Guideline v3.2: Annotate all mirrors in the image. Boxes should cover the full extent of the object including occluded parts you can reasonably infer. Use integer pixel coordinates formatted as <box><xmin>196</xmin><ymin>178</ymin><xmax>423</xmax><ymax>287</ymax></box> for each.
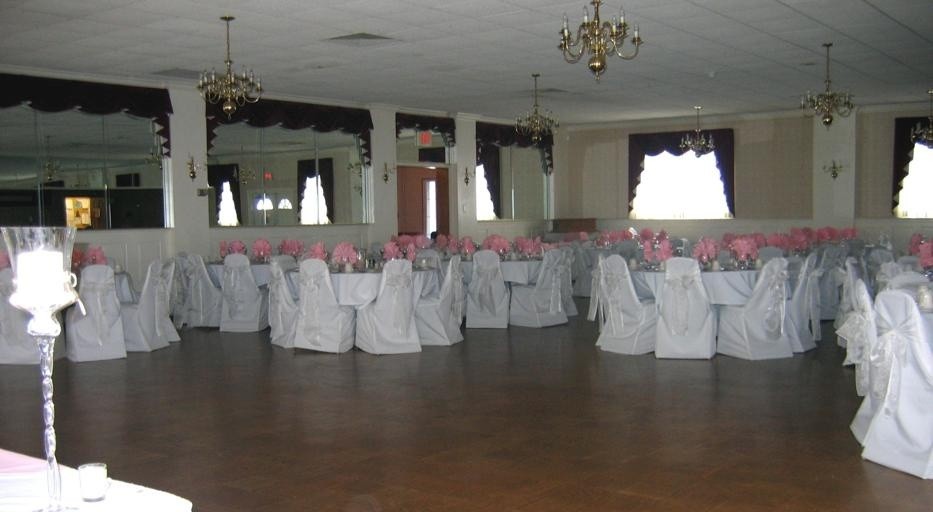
<box><xmin>473</xmin><ymin>118</ymin><xmax>551</xmax><ymax>220</ymax></box>
<box><xmin>203</xmin><ymin>90</ymin><xmax>377</xmax><ymax>229</ymax></box>
<box><xmin>0</xmin><ymin>71</ymin><xmax>174</xmax><ymax>233</ymax></box>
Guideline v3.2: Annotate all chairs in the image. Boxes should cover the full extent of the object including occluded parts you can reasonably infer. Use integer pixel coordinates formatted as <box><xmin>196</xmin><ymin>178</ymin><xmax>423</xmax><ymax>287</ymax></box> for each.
<box><xmin>175</xmin><ymin>234</ymin><xmax>589</xmax><ymax>353</ymax></box>
<box><xmin>585</xmin><ymin>224</ymin><xmax>855</xmax><ymax>361</ymax></box>
<box><xmin>833</xmin><ymin>232</ymin><xmax>933</xmax><ymax>479</ymax></box>
<box><xmin>1</xmin><ymin>246</ymin><xmax>177</xmax><ymax>365</ymax></box>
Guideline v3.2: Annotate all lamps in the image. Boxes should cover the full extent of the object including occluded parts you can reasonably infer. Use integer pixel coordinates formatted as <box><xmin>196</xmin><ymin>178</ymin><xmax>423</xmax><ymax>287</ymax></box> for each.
<box><xmin>796</xmin><ymin>36</ymin><xmax>863</xmax><ymax>136</ymax></box>
<box><xmin>672</xmin><ymin>103</ymin><xmax>722</xmax><ymax>161</ymax></box>
<box><xmin>188</xmin><ymin>12</ymin><xmax>268</xmax><ymax>125</ymax></box>
<box><xmin>40</xmin><ymin>134</ymin><xmax>60</xmax><ymax>183</ymax></box>
<box><xmin>255</xmin><ymin>189</ymin><xmax>275</xmax><ymax>226</ymax></box>
<box><xmin>546</xmin><ymin>2</ymin><xmax>648</xmax><ymax>93</ymax></box>
<box><xmin>520</xmin><ymin>72</ymin><xmax>564</xmax><ymax>146</ymax></box>
